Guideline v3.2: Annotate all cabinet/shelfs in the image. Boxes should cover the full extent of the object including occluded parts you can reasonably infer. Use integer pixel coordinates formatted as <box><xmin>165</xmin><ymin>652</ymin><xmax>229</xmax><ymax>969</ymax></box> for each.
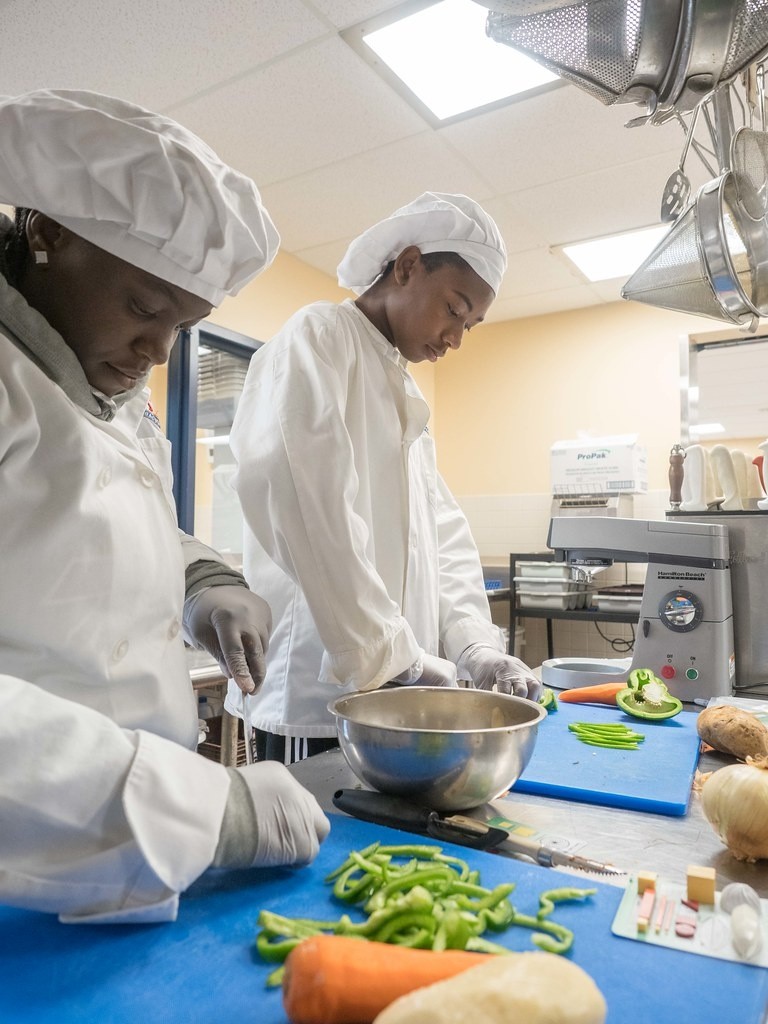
<box><xmin>509</xmin><ymin>552</ymin><xmax>640</xmax><ymax>658</ymax></box>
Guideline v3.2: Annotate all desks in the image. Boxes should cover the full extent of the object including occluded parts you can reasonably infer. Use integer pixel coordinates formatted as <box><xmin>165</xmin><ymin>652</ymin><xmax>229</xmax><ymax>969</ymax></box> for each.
<box><xmin>0</xmin><ymin>646</ymin><xmax>768</xmax><ymax>1024</ymax></box>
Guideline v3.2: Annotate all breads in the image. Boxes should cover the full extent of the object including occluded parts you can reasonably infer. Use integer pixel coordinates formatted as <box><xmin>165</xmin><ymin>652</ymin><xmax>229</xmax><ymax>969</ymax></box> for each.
<box><xmin>696</xmin><ymin>704</ymin><xmax>768</xmax><ymax>760</ymax></box>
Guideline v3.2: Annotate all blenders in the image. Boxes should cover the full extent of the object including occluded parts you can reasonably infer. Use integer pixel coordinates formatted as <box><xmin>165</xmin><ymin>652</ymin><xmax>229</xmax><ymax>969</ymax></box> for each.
<box><xmin>541</xmin><ymin>516</ymin><xmax>736</xmax><ymax>703</ymax></box>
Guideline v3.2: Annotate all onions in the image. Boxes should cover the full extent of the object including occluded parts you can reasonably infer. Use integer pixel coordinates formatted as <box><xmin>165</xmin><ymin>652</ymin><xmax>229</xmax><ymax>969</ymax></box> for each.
<box><xmin>700</xmin><ymin>754</ymin><xmax>768</xmax><ymax>862</ymax></box>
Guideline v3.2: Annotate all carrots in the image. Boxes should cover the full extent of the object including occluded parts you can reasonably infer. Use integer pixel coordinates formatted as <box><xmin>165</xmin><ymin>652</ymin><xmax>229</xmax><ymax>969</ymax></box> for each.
<box><xmin>282</xmin><ymin>935</ymin><xmax>516</xmax><ymax>1024</ymax></box>
<box><xmin>558</xmin><ymin>681</ymin><xmax>629</xmax><ymax>706</ymax></box>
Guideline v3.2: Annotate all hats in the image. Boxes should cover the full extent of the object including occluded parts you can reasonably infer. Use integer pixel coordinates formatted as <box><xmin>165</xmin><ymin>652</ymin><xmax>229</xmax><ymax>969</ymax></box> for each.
<box><xmin>336</xmin><ymin>191</ymin><xmax>509</xmax><ymax>299</ymax></box>
<box><xmin>0</xmin><ymin>88</ymin><xmax>280</xmax><ymax>308</ymax></box>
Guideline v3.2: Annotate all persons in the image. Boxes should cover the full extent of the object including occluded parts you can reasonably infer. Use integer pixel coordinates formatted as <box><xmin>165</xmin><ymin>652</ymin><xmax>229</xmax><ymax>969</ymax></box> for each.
<box><xmin>0</xmin><ymin>89</ymin><xmax>331</xmax><ymax>925</ymax></box>
<box><xmin>224</xmin><ymin>188</ymin><xmax>542</xmax><ymax>764</ymax></box>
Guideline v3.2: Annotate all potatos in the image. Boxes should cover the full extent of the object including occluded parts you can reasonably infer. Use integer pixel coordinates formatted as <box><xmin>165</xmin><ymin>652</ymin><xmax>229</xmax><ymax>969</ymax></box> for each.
<box><xmin>373</xmin><ymin>949</ymin><xmax>607</xmax><ymax>1024</ymax></box>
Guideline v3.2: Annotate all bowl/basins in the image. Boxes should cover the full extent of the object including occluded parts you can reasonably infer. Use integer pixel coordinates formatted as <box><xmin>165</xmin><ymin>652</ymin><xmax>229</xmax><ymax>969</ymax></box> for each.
<box><xmin>326</xmin><ymin>685</ymin><xmax>547</xmax><ymax>813</ymax></box>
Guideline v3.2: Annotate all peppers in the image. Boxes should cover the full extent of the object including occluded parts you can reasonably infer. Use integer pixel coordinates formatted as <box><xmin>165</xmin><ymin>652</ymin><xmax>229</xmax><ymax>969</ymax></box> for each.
<box><xmin>255</xmin><ymin>840</ymin><xmax>598</xmax><ymax>987</ymax></box>
<box><xmin>536</xmin><ymin>687</ymin><xmax>558</xmax><ymax>711</ymax></box>
<box><xmin>616</xmin><ymin>669</ymin><xmax>683</xmax><ymax>720</ymax></box>
<box><xmin>567</xmin><ymin>722</ymin><xmax>646</xmax><ymax>750</ymax></box>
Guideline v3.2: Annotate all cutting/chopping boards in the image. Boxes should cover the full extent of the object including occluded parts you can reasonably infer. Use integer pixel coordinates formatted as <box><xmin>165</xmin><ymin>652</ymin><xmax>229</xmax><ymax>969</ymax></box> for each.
<box><xmin>0</xmin><ymin>810</ymin><xmax>768</xmax><ymax>1024</ymax></box>
<box><xmin>508</xmin><ymin>693</ymin><xmax>701</xmax><ymax>818</ymax></box>
